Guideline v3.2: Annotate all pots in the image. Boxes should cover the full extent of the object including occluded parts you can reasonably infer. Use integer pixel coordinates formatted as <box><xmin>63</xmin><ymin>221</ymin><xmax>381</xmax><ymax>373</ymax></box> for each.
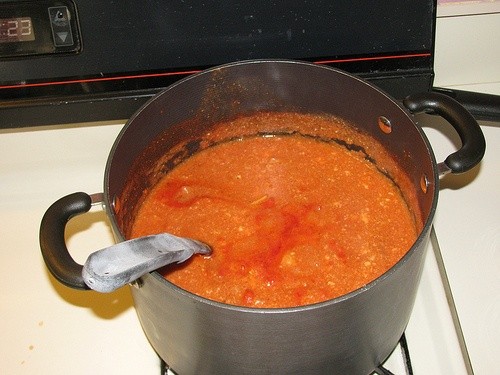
<box><xmin>38</xmin><ymin>55</ymin><xmax>487</xmax><ymax>374</ymax></box>
